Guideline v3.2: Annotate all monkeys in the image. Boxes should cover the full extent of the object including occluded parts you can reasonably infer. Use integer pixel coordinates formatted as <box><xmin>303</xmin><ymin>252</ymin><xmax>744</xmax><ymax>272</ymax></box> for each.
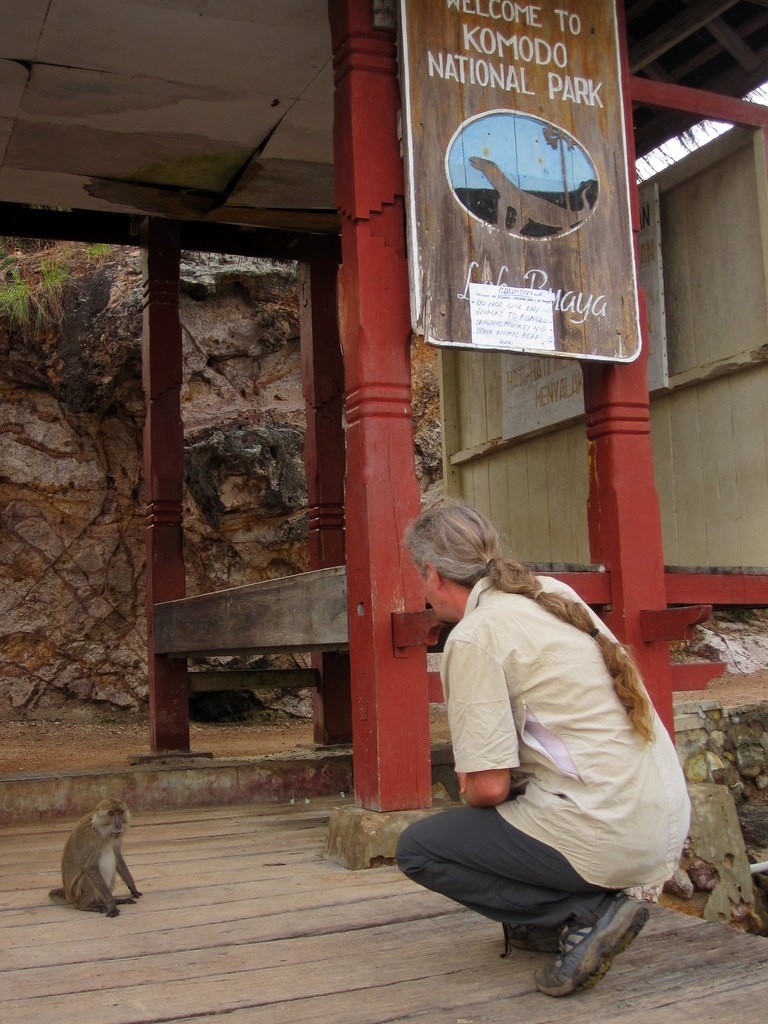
<box><xmin>49</xmin><ymin>798</ymin><xmax>143</xmax><ymax>918</ymax></box>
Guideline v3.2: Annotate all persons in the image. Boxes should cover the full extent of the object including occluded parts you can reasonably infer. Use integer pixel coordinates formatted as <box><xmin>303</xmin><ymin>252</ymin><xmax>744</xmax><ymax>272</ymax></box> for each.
<box><xmin>395</xmin><ymin>502</ymin><xmax>691</xmax><ymax>997</ymax></box>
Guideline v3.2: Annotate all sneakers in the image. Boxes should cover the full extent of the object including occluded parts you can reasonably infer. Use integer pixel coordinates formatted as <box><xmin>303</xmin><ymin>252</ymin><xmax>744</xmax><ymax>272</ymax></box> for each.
<box><xmin>508</xmin><ymin>920</ymin><xmax>563</xmax><ymax>952</ymax></box>
<box><xmin>533</xmin><ymin>891</ymin><xmax>650</xmax><ymax>997</ymax></box>
